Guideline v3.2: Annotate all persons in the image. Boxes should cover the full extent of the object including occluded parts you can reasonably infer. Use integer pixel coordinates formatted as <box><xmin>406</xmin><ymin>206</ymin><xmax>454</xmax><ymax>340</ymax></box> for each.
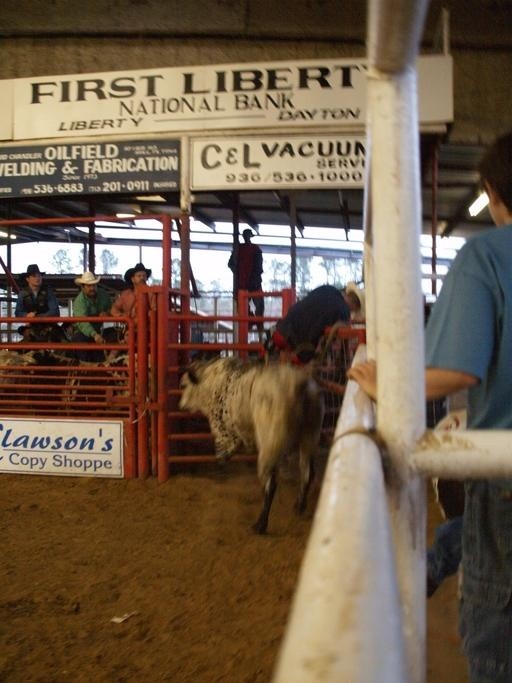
<box><xmin>345</xmin><ymin>130</ymin><xmax>512</xmax><ymax>683</ymax></box>
<box><xmin>272</xmin><ymin>281</ymin><xmax>364</xmax><ymax>367</ymax></box>
<box><xmin>110</xmin><ymin>263</ymin><xmax>156</xmax><ymax>315</ymax></box>
<box><xmin>228</xmin><ymin>229</ymin><xmax>264</xmax><ymax>329</ymax></box>
<box><xmin>14</xmin><ymin>265</ymin><xmax>60</xmax><ymax>317</ymax></box>
<box><xmin>72</xmin><ymin>272</ymin><xmax>112</xmax><ymax>344</ymax></box>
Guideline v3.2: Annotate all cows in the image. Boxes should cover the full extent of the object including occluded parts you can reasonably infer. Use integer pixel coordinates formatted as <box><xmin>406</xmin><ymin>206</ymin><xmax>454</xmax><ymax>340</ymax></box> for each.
<box><xmin>23</xmin><ymin>350</ymin><xmax>149</xmax><ymax>416</ymax></box>
<box><xmin>178</xmin><ymin>322</ymin><xmax>345</xmax><ymax>538</ymax></box>
<box><xmin>0</xmin><ymin>350</ymin><xmax>23</xmax><ymax>385</ymax></box>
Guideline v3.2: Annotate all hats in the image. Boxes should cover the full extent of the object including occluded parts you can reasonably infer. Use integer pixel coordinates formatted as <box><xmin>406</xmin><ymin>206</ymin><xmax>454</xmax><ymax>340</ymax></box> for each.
<box><xmin>346</xmin><ymin>282</ymin><xmax>366</xmax><ymax>322</ymax></box>
<box><xmin>124</xmin><ymin>262</ymin><xmax>152</xmax><ymax>283</ymax></box>
<box><xmin>22</xmin><ymin>263</ymin><xmax>45</xmax><ymax>278</ymax></box>
<box><xmin>75</xmin><ymin>271</ymin><xmax>101</xmax><ymax>287</ymax></box>
